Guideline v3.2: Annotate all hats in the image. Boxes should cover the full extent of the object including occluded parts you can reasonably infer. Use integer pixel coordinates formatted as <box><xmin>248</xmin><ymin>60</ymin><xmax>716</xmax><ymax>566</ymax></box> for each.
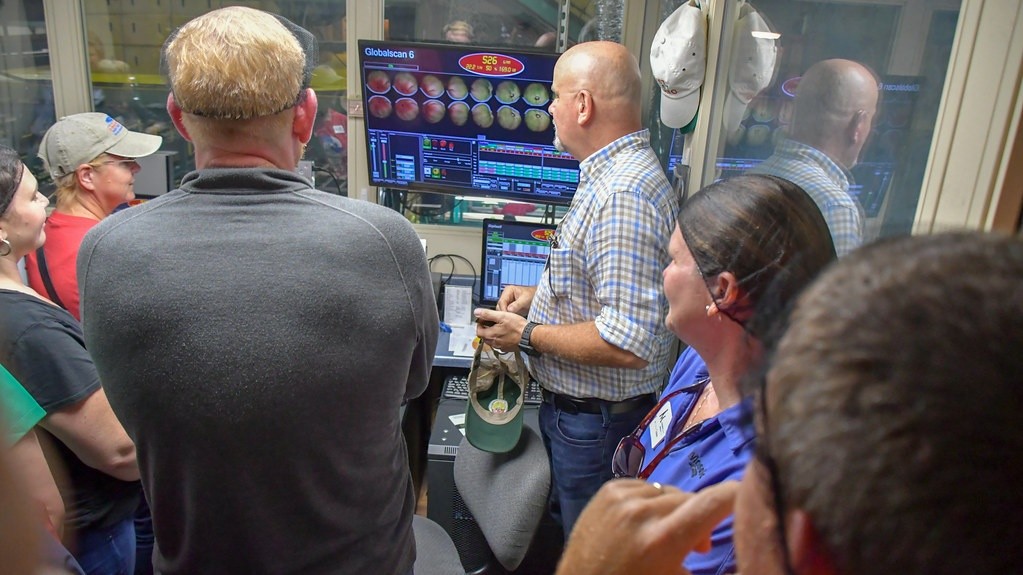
<box><xmin>465</xmin><ymin>337</ymin><xmax>530</xmax><ymax>454</ymax></box>
<box><xmin>649</xmin><ymin>0</ymin><xmax>708</xmax><ymax>135</ymax></box>
<box><xmin>37</xmin><ymin>113</ymin><xmax>163</xmax><ymax>179</ymax></box>
<box><xmin>723</xmin><ymin>2</ymin><xmax>777</xmax><ymax>131</ymax></box>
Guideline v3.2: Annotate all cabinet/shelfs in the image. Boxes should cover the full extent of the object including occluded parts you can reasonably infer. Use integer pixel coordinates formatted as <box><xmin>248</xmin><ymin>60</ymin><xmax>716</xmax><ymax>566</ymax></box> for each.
<box><xmin>82</xmin><ymin>0</ymin><xmax>222</xmax><ymax>73</ymax></box>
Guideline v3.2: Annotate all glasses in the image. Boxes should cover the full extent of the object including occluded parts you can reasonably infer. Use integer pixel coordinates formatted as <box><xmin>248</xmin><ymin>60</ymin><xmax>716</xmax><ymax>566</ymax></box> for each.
<box><xmin>57</xmin><ymin>157</ymin><xmax>136</xmax><ymax>179</ymax></box>
<box><xmin>612</xmin><ymin>378</ymin><xmax>707</xmax><ymax>480</ymax></box>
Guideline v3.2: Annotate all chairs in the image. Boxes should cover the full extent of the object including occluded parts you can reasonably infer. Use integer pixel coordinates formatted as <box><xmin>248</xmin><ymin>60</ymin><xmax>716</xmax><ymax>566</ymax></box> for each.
<box><xmin>411</xmin><ymin>424</ymin><xmax>551</xmax><ymax>575</ymax></box>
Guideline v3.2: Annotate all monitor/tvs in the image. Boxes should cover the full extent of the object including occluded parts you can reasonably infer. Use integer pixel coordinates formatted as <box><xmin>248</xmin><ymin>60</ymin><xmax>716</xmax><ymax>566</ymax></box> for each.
<box><xmin>666</xmin><ymin>71</ymin><xmax>927</xmax><ymax>221</ymax></box>
<box><xmin>358</xmin><ymin>39</ymin><xmax>580</xmax><ymax>205</ymax></box>
<box><xmin>480</xmin><ymin>218</ymin><xmax>558</xmax><ymax>308</ymax></box>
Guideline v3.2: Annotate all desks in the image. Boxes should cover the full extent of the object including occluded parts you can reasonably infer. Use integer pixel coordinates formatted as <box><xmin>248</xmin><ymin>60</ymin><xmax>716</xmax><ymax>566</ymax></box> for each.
<box><xmin>0</xmin><ymin>64</ymin><xmax>170</xmax><ymax>89</ymax></box>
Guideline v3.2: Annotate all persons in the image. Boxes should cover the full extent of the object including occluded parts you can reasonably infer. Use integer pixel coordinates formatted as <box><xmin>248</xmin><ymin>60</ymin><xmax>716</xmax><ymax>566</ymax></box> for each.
<box><xmin>78</xmin><ymin>5</ymin><xmax>440</xmax><ymax>575</ymax></box>
<box><xmin>557</xmin><ymin>229</ymin><xmax>1023</xmax><ymax>575</ymax></box>
<box><xmin>474</xmin><ymin>41</ymin><xmax>680</xmax><ymax>575</ymax></box>
<box><xmin>24</xmin><ymin>112</ymin><xmax>163</xmax><ymax>320</ymax></box>
<box><xmin>629</xmin><ymin>173</ymin><xmax>839</xmax><ymax>575</ymax></box>
<box><xmin>88</xmin><ymin>31</ymin><xmax>108</xmax><ymax>109</ymax></box>
<box><xmin>752</xmin><ymin>58</ymin><xmax>879</xmax><ymax>256</ymax></box>
<box><xmin>443</xmin><ymin>21</ymin><xmax>474</xmax><ymax>42</ymax></box>
<box><xmin>0</xmin><ymin>145</ymin><xmax>142</xmax><ymax>575</ymax></box>
<box><xmin>0</xmin><ymin>364</ymin><xmax>66</xmax><ymax>540</ymax></box>
<box><xmin>314</xmin><ymin>95</ymin><xmax>347</xmax><ymax>176</ymax></box>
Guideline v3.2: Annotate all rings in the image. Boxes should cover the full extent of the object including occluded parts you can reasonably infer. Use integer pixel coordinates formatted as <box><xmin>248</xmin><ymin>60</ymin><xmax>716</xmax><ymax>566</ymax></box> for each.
<box><xmin>653</xmin><ymin>482</ymin><xmax>665</xmax><ymax>495</ymax></box>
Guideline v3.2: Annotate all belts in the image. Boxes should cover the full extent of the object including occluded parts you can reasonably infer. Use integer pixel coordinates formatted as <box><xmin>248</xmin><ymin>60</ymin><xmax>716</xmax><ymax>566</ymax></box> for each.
<box><xmin>539</xmin><ymin>384</ymin><xmax>656</xmax><ymax>416</ymax></box>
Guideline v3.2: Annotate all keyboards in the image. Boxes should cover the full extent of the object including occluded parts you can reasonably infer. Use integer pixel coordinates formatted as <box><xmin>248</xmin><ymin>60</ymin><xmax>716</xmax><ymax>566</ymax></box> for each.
<box><xmin>443</xmin><ymin>377</ymin><xmax>543</xmax><ymax>404</ymax></box>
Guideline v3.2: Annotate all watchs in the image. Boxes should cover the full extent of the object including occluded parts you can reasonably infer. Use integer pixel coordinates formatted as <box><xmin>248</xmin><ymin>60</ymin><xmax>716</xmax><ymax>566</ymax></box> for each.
<box><xmin>518</xmin><ymin>321</ymin><xmax>543</xmax><ymax>357</ymax></box>
